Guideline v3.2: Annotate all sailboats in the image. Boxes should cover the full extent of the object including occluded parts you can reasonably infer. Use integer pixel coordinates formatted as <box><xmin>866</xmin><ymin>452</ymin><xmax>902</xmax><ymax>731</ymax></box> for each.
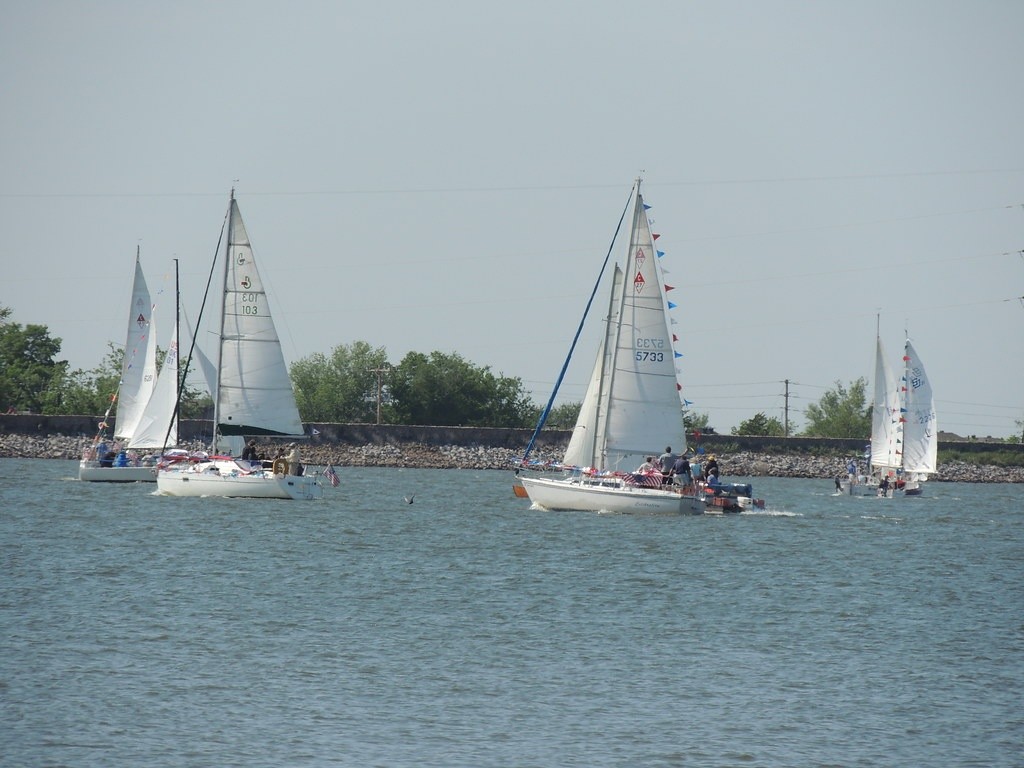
<box><xmin>515</xmin><ymin>173</ymin><xmax>765</xmax><ymax>518</ymax></box>
<box><xmin>835</xmin><ymin>314</ymin><xmax>939</xmax><ymax>497</ymax></box>
<box><xmin>77</xmin><ymin>243</ymin><xmax>217</xmax><ymax>480</ymax></box>
<box><xmin>155</xmin><ymin>188</ymin><xmax>319</xmax><ymax>499</ymax></box>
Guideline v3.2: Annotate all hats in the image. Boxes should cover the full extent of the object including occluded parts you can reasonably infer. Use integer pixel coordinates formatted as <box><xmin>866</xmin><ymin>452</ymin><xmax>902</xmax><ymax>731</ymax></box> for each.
<box><xmin>708</xmin><ymin>456</ymin><xmax>715</xmax><ymax>460</ymax></box>
<box><xmin>100</xmin><ymin>437</ymin><xmax>108</xmax><ymax>443</ymax></box>
<box><xmin>693</xmin><ymin>458</ymin><xmax>700</xmax><ymax>463</ymax></box>
<box><xmin>289</xmin><ymin>442</ymin><xmax>297</xmax><ymax>447</ymax></box>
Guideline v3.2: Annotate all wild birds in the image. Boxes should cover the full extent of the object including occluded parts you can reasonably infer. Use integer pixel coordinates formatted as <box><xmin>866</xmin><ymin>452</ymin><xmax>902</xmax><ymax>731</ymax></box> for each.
<box><xmin>404</xmin><ymin>495</ymin><xmax>414</xmax><ymax>504</ymax></box>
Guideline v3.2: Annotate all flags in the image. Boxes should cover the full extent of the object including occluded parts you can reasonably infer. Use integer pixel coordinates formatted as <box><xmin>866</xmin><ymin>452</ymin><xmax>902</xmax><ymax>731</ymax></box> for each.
<box><xmin>323</xmin><ymin>465</ymin><xmax>340</xmax><ymax>487</ymax></box>
<box><xmin>620</xmin><ymin>473</ymin><xmax>662</xmax><ymax>488</ymax></box>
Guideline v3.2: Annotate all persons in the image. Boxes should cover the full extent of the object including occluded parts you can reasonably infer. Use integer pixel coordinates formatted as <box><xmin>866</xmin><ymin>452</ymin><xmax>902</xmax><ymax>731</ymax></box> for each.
<box><xmin>637</xmin><ymin>447</ymin><xmax>719</xmax><ymax>495</ymax></box>
<box><xmin>0</xmin><ymin>432</ymin><xmax>1024</xmax><ymax>482</ymax></box>
<box><xmin>833</xmin><ymin>460</ymin><xmax>903</xmax><ymax>496</ymax></box>
